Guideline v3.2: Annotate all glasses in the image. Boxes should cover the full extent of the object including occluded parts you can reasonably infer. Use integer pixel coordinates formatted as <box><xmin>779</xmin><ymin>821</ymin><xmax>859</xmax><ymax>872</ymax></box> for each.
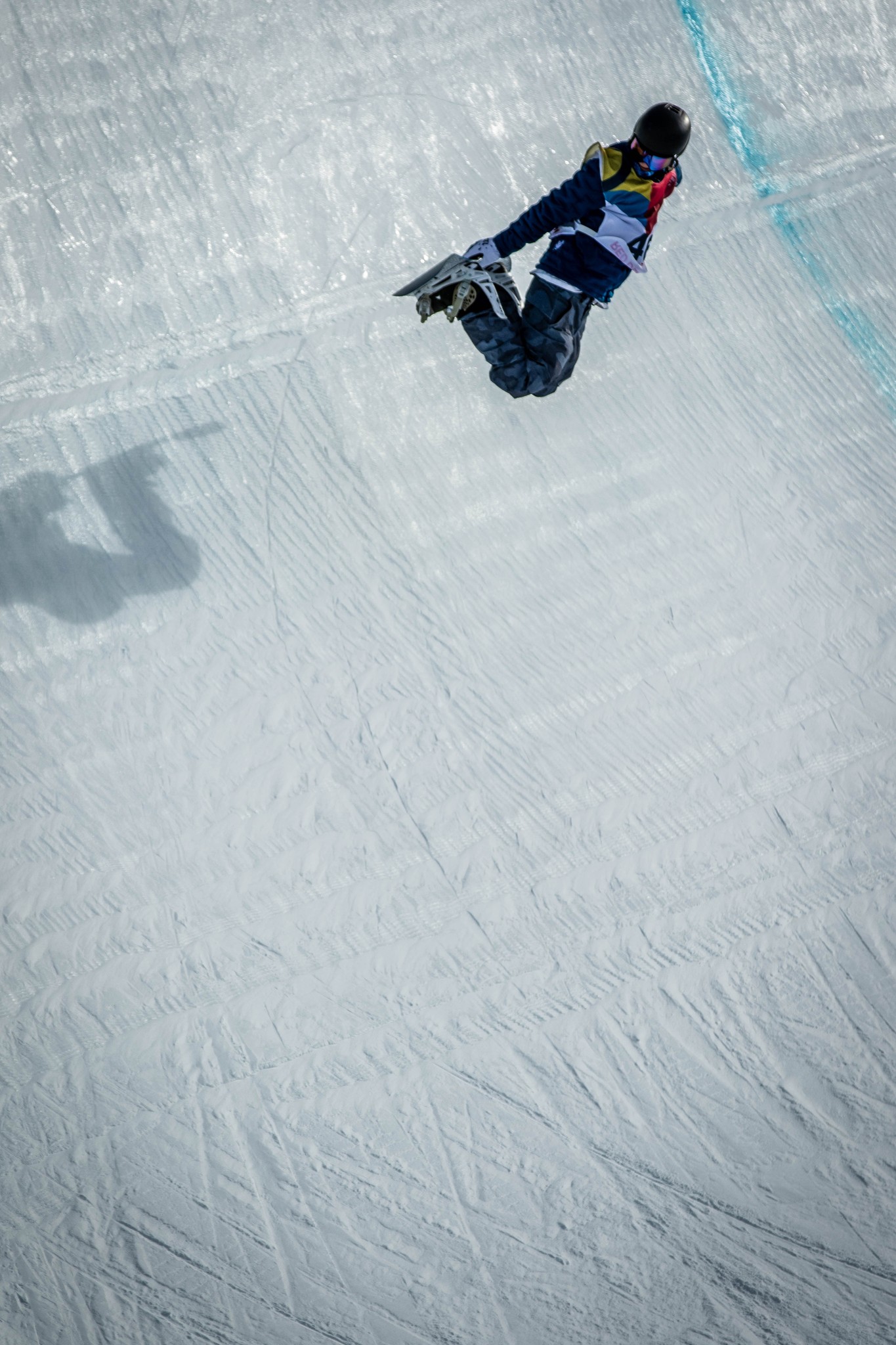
<box><xmin>629</xmin><ymin>133</ymin><xmax>674</xmax><ymax>171</ymax></box>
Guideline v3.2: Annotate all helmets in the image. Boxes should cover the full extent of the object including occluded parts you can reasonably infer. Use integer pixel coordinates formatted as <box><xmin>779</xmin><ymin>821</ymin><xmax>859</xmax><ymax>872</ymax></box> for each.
<box><xmin>627</xmin><ymin>102</ymin><xmax>691</xmax><ymax>180</ymax></box>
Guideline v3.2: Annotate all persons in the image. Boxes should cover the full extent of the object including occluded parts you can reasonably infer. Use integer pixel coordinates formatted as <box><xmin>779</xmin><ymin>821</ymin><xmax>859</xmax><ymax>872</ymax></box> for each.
<box><xmin>416</xmin><ymin>102</ymin><xmax>691</xmax><ymax>399</ymax></box>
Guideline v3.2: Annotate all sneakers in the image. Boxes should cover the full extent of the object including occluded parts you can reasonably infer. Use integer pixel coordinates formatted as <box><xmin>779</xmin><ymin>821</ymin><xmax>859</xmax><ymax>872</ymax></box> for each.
<box><xmin>416</xmin><ymin>256</ymin><xmax>508</xmax><ymax>319</ymax></box>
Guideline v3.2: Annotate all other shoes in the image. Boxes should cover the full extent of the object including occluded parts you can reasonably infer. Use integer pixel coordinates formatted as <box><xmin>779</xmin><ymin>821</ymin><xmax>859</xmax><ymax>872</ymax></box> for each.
<box><xmin>482</xmin><ymin>261</ymin><xmax>522</xmax><ymax>320</ymax></box>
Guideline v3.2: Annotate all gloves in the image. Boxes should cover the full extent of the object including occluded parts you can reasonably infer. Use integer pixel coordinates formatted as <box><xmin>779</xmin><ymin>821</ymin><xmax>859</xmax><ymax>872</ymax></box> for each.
<box><xmin>464</xmin><ymin>237</ymin><xmax>500</xmax><ymax>268</ymax></box>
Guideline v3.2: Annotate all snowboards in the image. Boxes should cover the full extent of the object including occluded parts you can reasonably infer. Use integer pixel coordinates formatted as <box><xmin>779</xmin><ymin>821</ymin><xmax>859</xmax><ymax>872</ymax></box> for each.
<box><xmin>391</xmin><ymin>252</ymin><xmax>512</xmax><ymax>297</ymax></box>
